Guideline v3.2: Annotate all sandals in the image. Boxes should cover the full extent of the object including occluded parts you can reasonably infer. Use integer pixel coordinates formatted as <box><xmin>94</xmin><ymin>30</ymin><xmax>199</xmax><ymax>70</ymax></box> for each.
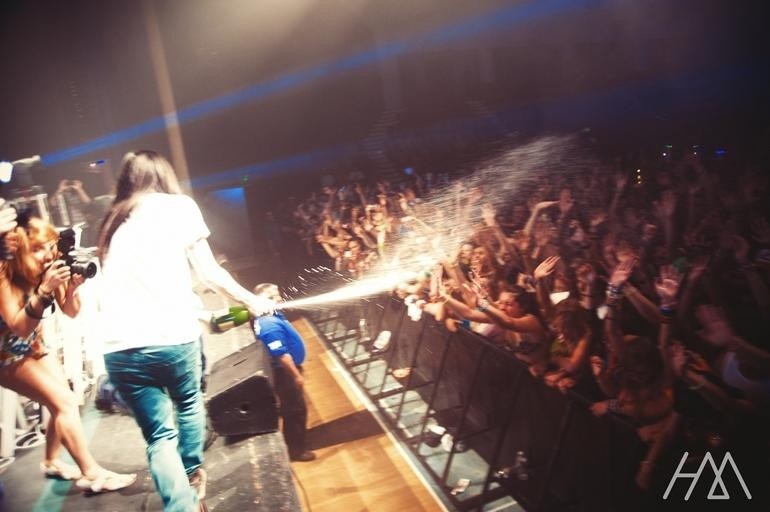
<box><xmin>39</xmin><ymin>459</ymin><xmax>78</xmax><ymax>481</ymax></box>
<box><xmin>77</xmin><ymin>468</ymin><xmax>139</xmax><ymax>492</ymax></box>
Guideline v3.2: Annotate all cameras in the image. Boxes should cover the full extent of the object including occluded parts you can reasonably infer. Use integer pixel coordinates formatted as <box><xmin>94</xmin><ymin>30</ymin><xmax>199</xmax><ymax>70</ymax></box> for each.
<box><xmin>56</xmin><ymin>229</ymin><xmax>97</xmax><ymax>278</ymax></box>
<box><xmin>10</xmin><ymin>201</ymin><xmax>32</xmax><ymax>226</ymax></box>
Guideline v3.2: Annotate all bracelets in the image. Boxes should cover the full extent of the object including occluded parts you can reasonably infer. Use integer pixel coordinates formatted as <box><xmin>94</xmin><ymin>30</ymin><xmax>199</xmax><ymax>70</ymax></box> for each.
<box><xmin>38</xmin><ymin>284</ymin><xmax>52</xmax><ymax>296</ymax></box>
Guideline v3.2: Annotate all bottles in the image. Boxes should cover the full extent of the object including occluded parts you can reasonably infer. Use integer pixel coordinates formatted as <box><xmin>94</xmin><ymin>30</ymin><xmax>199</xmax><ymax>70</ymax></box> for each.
<box><xmin>514</xmin><ymin>450</ymin><xmax>530</xmax><ymax>483</ymax></box>
<box><xmin>210</xmin><ymin>296</ymin><xmax>277</xmax><ymax>332</ymax></box>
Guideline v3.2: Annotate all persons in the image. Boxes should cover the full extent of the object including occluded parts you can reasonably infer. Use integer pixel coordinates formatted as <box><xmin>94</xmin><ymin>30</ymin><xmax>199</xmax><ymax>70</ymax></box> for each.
<box><xmin>0</xmin><ymin>197</ymin><xmax>22</xmax><ymax>262</ymax></box>
<box><xmin>46</xmin><ymin>177</ymin><xmax>93</xmax><ymax>229</ymax></box>
<box><xmin>1</xmin><ymin>215</ymin><xmax>140</xmax><ymax>497</ymax></box>
<box><xmin>248</xmin><ymin>100</ymin><xmax>770</xmax><ymax>490</ymax></box>
<box><xmin>85</xmin><ymin>147</ymin><xmax>278</xmax><ymax>512</ymax></box>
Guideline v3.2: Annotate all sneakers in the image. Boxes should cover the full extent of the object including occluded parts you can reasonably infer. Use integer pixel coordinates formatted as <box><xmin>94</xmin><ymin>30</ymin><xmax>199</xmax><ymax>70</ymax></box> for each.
<box><xmin>290</xmin><ymin>449</ymin><xmax>315</xmax><ymax>462</ymax></box>
<box><xmin>189</xmin><ymin>468</ymin><xmax>206</xmax><ymax>501</ymax></box>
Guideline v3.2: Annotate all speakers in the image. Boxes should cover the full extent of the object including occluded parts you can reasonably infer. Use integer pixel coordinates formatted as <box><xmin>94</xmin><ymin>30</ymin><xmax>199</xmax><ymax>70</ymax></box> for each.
<box><xmin>203</xmin><ymin>341</ymin><xmax>278</xmax><ymax>434</ymax></box>
<box><xmin>203</xmin><ymin>444</ymin><xmax>301</xmax><ymax>512</ymax></box>
